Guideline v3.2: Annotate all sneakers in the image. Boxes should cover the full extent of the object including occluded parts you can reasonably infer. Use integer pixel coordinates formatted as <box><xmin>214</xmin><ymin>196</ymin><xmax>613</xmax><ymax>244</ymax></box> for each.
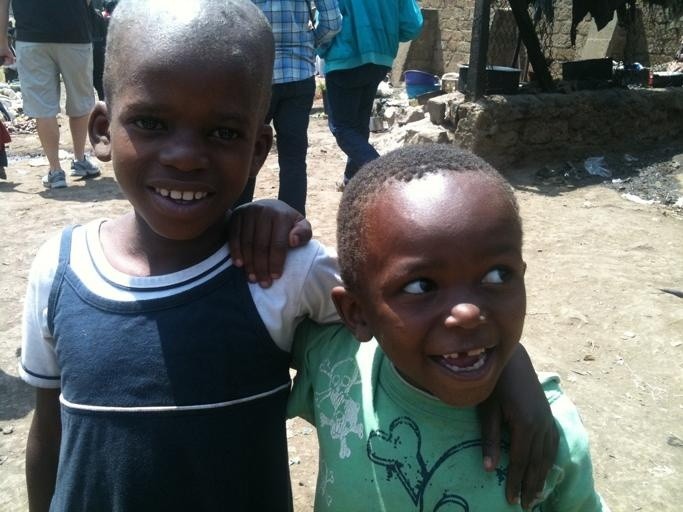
<box><xmin>69</xmin><ymin>155</ymin><xmax>101</xmax><ymax>178</ymax></box>
<box><xmin>42</xmin><ymin>169</ymin><xmax>67</xmax><ymax>188</ymax></box>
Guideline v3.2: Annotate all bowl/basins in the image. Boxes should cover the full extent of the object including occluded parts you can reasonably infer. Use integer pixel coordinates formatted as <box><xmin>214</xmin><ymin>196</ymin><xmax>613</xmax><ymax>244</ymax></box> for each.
<box><xmin>402</xmin><ymin>70</ymin><xmax>440</xmax><ymax>99</ymax></box>
<box><xmin>560</xmin><ymin>57</ymin><xmax>613</xmax><ymax>81</ymax></box>
<box><xmin>458</xmin><ymin>63</ymin><xmax>523</xmax><ymax>94</ymax></box>
<box><xmin>442</xmin><ymin>79</ymin><xmax>458</xmax><ymax>94</ymax></box>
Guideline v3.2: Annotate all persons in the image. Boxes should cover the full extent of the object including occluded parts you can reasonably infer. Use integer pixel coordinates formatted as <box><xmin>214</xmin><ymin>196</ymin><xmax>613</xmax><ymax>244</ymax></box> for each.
<box><xmin>14</xmin><ymin>1</ymin><xmax>561</xmax><ymax>511</ymax></box>
<box><xmin>314</xmin><ymin>0</ymin><xmax>424</xmax><ymax>192</ymax></box>
<box><xmin>228</xmin><ymin>1</ymin><xmax>343</xmax><ymax>219</ymax></box>
<box><xmin>227</xmin><ymin>142</ymin><xmax>606</xmax><ymax>511</ymax></box>
<box><xmin>0</xmin><ymin>0</ymin><xmax>119</xmax><ymax>192</ymax></box>
